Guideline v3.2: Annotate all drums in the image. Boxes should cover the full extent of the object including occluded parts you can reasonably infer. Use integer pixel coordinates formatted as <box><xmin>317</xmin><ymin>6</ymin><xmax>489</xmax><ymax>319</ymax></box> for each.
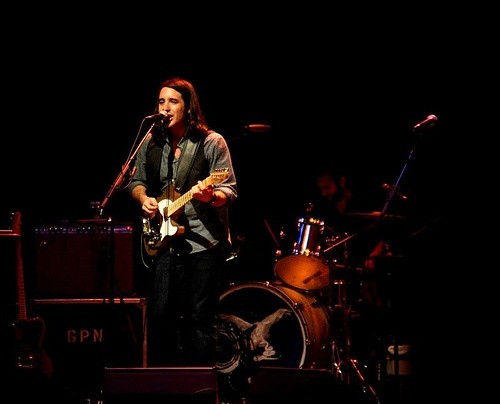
<box><xmin>218</xmin><ymin>281</ymin><xmax>330</xmax><ymax>369</ymax></box>
<box><xmin>274</xmin><ymin>218</ymin><xmax>336</xmax><ymax>292</ymax></box>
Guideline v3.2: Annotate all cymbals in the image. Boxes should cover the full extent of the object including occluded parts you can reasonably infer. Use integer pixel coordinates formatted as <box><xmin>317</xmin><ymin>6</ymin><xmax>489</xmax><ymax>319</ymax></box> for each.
<box><xmin>337</xmin><ymin>209</ymin><xmax>401</xmax><ymax>226</ymax></box>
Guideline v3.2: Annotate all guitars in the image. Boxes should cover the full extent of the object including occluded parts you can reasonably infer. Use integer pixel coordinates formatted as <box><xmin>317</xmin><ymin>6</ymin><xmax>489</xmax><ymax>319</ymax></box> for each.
<box><xmin>4</xmin><ymin>210</ymin><xmax>46</xmax><ymax>372</ymax></box>
<box><xmin>140</xmin><ymin>167</ymin><xmax>228</xmax><ymax>256</ymax></box>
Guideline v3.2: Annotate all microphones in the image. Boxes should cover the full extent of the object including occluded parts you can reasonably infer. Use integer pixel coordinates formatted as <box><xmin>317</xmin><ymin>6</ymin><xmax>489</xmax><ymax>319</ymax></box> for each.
<box><xmin>145</xmin><ymin>110</ymin><xmax>167</xmax><ymax>119</ymax></box>
<box><xmin>413</xmin><ymin>114</ymin><xmax>438</xmax><ymax>128</ymax></box>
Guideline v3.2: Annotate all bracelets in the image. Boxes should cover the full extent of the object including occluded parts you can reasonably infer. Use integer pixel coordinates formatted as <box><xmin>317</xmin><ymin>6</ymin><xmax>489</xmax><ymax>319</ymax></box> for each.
<box><xmin>207</xmin><ymin>194</ymin><xmax>218</xmax><ymax>203</ymax></box>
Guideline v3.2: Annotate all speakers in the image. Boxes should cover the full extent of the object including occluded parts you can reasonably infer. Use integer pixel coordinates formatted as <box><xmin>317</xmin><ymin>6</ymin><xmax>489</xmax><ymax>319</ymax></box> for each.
<box><xmin>103</xmin><ymin>364</ymin><xmax>224</xmax><ymax>404</ymax></box>
<box><xmin>28</xmin><ymin>231</ymin><xmax>135</xmax><ymax>300</ymax></box>
<box><xmin>249</xmin><ymin>366</ymin><xmax>371</xmax><ymax>404</ymax></box>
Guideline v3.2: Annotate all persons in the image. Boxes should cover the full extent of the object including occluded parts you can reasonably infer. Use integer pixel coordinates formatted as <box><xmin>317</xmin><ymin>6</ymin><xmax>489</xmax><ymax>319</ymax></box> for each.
<box><xmin>126</xmin><ymin>78</ymin><xmax>237</xmax><ymax>362</ymax></box>
<box><xmin>310</xmin><ymin>163</ymin><xmax>378</xmax><ymax>234</ymax></box>
